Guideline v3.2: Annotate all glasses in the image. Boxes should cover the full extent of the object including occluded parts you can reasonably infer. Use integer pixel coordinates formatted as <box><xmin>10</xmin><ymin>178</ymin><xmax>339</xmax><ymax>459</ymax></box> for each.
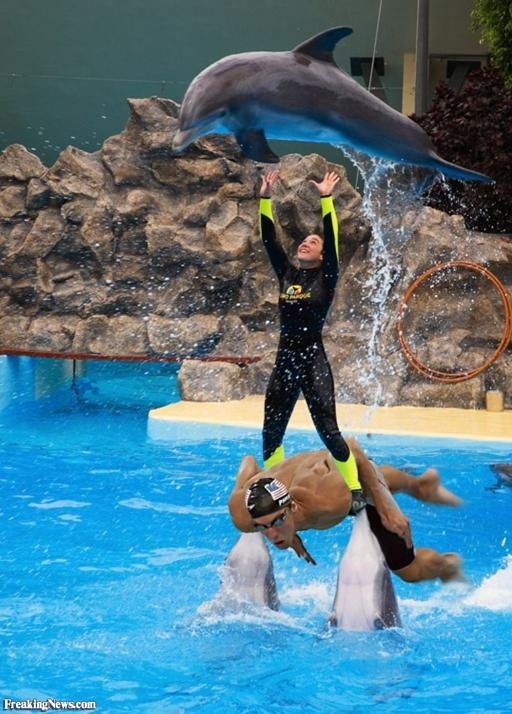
<box><xmin>253</xmin><ymin>509</ymin><xmax>288</xmax><ymax>533</ymax></box>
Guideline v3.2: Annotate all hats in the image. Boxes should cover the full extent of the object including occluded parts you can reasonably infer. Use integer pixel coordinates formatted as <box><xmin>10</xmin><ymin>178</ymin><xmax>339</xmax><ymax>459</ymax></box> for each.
<box><xmin>245</xmin><ymin>477</ymin><xmax>291</xmax><ymax>517</ymax></box>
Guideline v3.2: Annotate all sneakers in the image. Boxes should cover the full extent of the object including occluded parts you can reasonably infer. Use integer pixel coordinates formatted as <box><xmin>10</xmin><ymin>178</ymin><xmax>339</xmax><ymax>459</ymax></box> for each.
<box><xmin>349</xmin><ymin>489</ymin><xmax>365</xmax><ymax>515</ymax></box>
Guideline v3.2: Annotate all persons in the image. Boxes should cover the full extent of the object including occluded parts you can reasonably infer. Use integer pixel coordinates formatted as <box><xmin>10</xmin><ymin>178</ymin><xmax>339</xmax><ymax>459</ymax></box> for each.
<box><xmin>257</xmin><ymin>169</ymin><xmax>367</xmax><ymax>516</ymax></box>
<box><xmin>226</xmin><ymin>435</ymin><xmax>468</xmax><ymax>585</ymax></box>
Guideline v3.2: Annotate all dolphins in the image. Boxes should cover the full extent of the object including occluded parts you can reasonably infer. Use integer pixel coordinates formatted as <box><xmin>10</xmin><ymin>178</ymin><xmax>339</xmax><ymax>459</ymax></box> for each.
<box><xmin>489</xmin><ymin>463</ymin><xmax>511</xmax><ymax>488</ymax></box>
<box><xmin>170</xmin><ymin>23</ymin><xmax>497</xmax><ymax>186</ymax></box>
<box><xmin>218</xmin><ymin>529</ymin><xmax>279</xmax><ymax>618</ymax></box>
<box><xmin>323</xmin><ymin>506</ymin><xmax>402</xmax><ymax>632</ymax></box>
